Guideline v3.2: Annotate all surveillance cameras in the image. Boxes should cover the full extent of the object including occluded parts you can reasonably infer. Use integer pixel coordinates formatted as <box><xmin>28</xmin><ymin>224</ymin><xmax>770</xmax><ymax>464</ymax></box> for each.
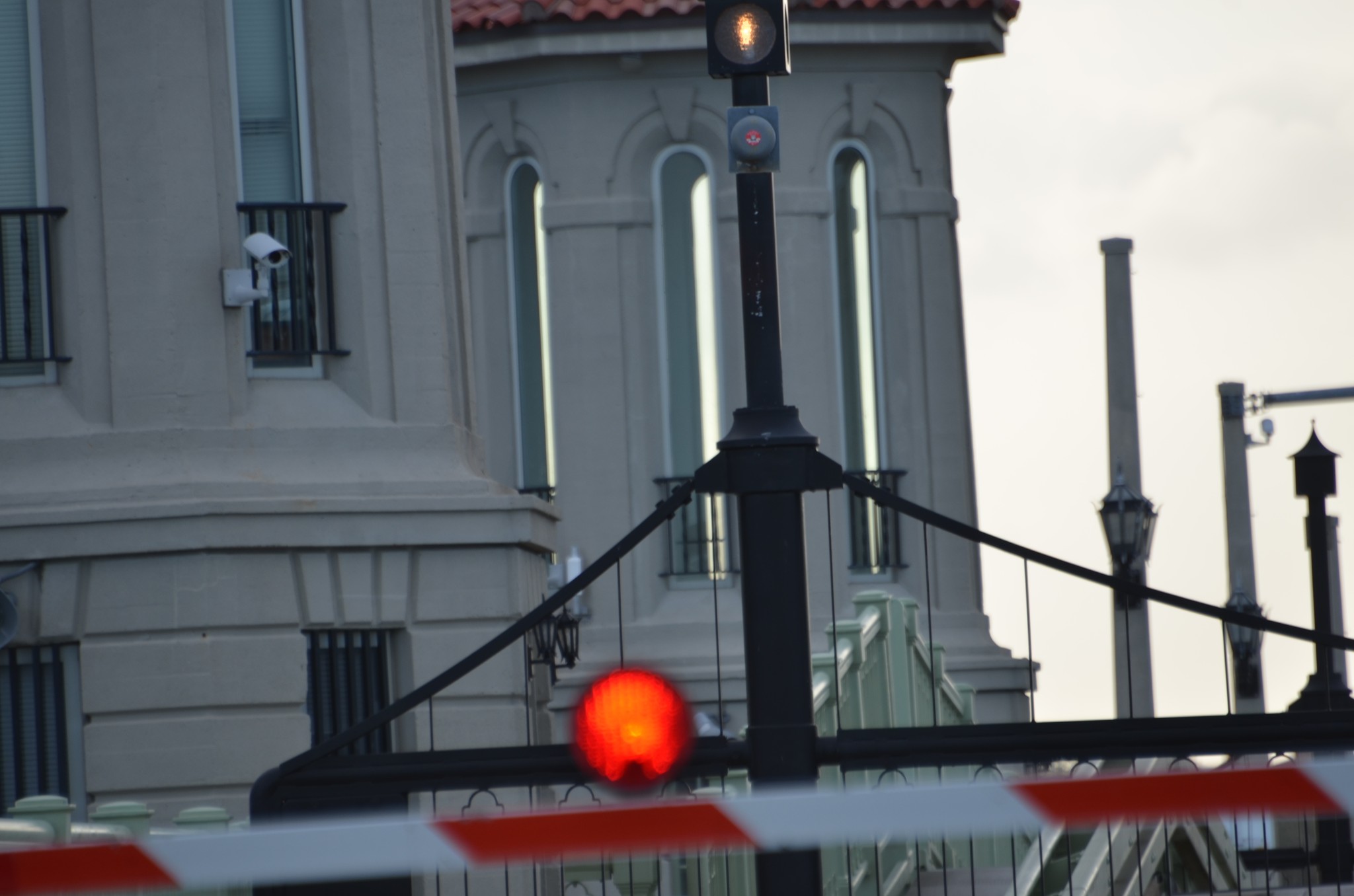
<box><xmin>242</xmin><ymin>232</ymin><xmax>292</xmax><ymax>268</ymax></box>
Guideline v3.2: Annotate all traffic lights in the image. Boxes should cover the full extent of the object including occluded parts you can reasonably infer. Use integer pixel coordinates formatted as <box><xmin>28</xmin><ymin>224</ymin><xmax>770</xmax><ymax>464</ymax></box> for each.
<box><xmin>703</xmin><ymin>1</ymin><xmax>795</xmax><ymax>73</ymax></box>
